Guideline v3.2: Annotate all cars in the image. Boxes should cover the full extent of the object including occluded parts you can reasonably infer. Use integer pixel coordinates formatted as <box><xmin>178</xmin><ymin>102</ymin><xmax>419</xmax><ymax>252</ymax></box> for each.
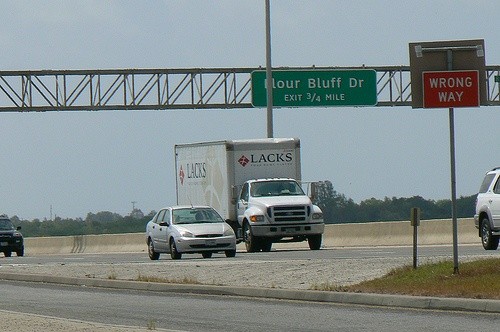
<box><xmin>146</xmin><ymin>206</ymin><xmax>236</xmax><ymax>260</ymax></box>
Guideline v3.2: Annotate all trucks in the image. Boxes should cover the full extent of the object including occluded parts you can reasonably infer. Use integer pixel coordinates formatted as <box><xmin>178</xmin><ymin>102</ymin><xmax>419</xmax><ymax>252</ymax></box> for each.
<box><xmin>174</xmin><ymin>138</ymin><xmax>325</xmax><ymax>252</ymax></box>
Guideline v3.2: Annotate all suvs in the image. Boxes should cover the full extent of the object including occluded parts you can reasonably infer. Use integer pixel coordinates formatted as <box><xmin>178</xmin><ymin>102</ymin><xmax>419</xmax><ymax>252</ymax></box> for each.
<box><xmin>0</xmin><ymin>215</ymin><xmax>24</xmax><ymax>256</ymax></box>
<box><xmin>474</xmin><ymin>167</ymin><xmax>500</xmax><ymax>250</ymax></box>
